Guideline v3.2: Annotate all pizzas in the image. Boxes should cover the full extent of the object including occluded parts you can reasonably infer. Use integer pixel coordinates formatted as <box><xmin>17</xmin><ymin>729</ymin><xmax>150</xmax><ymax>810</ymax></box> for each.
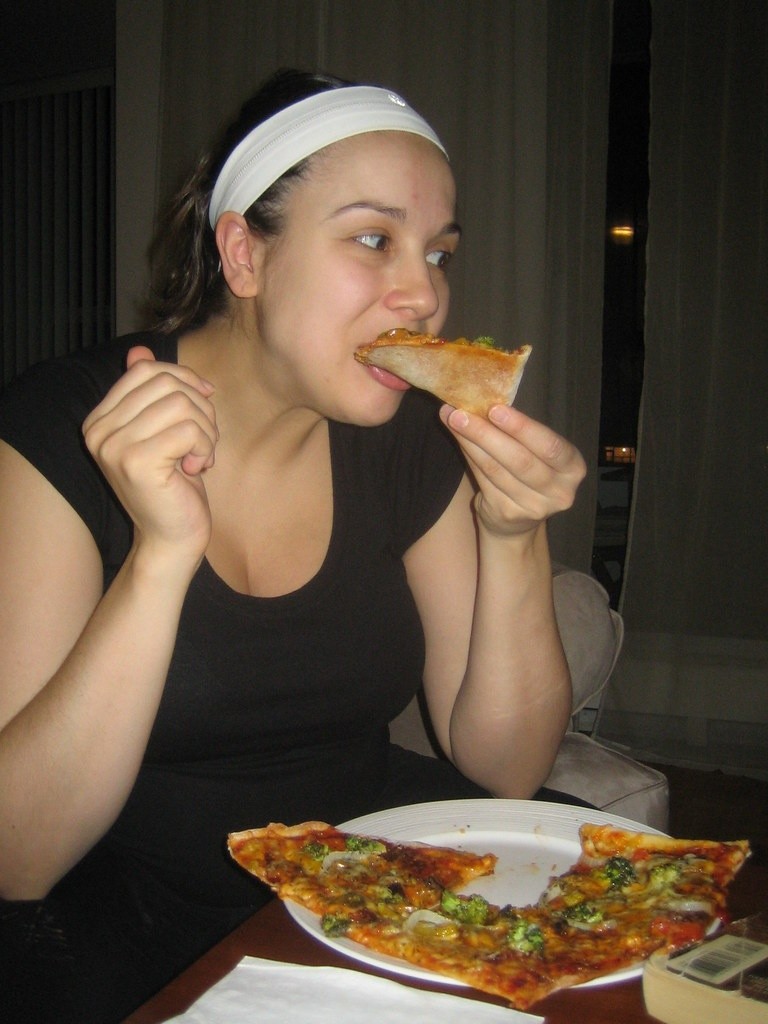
<box><xmin>354</xmin><ymin>328</ymin><xmax>532</xmax><ymax>419</ymax></box>
<box><xmin>228</xmin><ymin>821</ymin><xmax>751</xmax><ymax>1011</ymax></box>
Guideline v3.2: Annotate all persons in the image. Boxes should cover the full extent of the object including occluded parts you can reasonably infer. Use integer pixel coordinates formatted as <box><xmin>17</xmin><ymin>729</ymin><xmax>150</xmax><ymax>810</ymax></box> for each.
<box><xmin>0</xmin><ymin>66</ymin><xmax>604</xmax><ymax>1024</ymax></box>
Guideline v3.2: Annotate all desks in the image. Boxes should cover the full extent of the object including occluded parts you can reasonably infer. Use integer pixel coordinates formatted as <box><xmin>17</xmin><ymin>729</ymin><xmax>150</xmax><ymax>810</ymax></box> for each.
<box><xmin>123</xmin><ymin>864</ymin><xmax>768</xmax><ymax>1024</ymax></box>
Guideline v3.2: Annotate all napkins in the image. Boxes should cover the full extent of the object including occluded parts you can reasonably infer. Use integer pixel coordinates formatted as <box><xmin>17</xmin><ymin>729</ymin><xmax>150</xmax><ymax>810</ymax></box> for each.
<box><xmin>159</xmin><ymin>956</ymin><xmax>549</xmax><ymax>1024</ymax></box>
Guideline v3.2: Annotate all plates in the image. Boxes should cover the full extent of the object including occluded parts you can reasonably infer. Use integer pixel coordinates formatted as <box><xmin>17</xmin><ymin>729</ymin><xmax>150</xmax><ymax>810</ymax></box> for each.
<box><xmin>286</xmin><ymin>799</ymin><xmax>722</xmax><ymax>987</ymax></box>
